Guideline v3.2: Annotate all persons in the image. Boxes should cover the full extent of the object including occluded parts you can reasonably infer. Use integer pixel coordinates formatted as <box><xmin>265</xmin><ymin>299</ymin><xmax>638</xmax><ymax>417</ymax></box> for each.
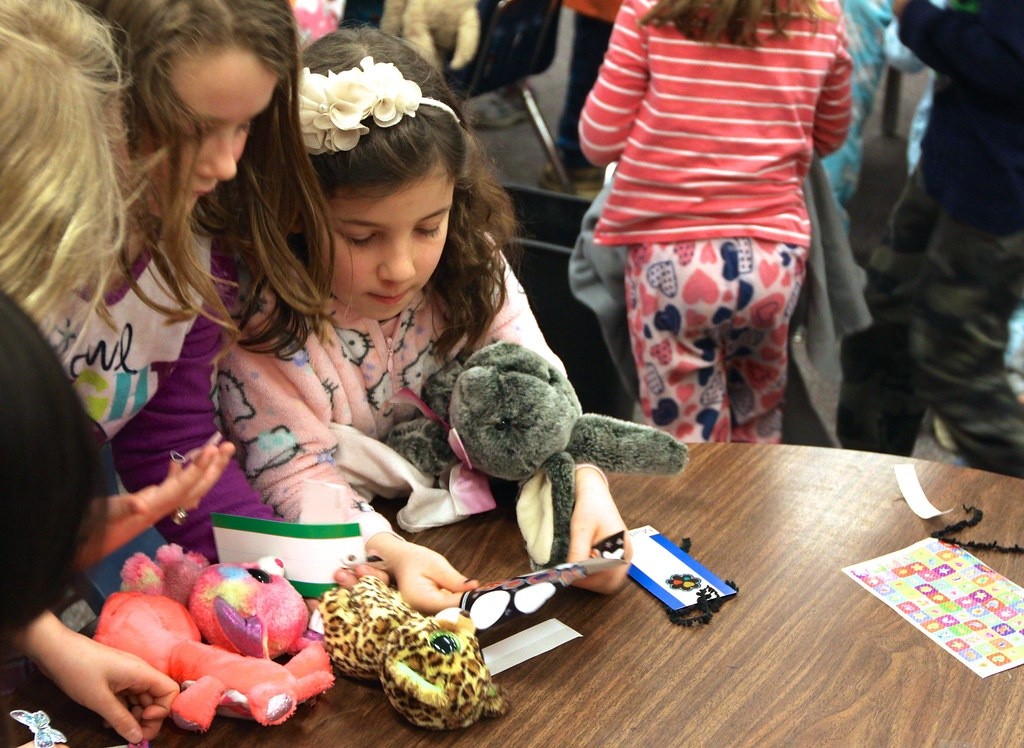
<box><xmin>0</xmin><ymin>0</ymin><xmax>1024</xmax><ymax>748</ymax></box>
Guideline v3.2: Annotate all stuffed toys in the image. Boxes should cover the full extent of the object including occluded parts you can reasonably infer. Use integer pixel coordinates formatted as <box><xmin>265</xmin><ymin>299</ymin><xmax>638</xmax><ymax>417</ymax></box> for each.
<box><xmin>386</xmin><ymin>340</ymin><xmax>688</xmax><ymax>569</ymax></box>
<box><xmin>93</xmin><ymin>543</ymin><xmax>335</xmax><ymax>733</ymax></box>
<box><xmin>319</xmin><ymin>576</ymin><xmax>510</xmax><ymax>731</ymax></box>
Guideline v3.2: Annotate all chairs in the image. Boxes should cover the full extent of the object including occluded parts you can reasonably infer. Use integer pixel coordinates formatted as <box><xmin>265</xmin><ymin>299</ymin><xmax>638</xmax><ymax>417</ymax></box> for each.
<box><xmin>842</xmin><ymin>133</ymin><xmax>912</xmax><ymax>280</ymax></box>
<box><xmin>448</xmin><ymin>0</ymin><xmax>576</xmax><ymax>193</ymax></box>
<box><xmin>498</xmin><ymin>186</ymin><xmax>614</xmax><ymax>418</ymax></box>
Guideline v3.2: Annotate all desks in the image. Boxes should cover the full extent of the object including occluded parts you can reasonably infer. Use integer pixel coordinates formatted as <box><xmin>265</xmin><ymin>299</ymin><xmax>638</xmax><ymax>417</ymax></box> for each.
<box><xmin>0</xmin><ymin>442</ymin><xmax>1024</xmax><ymax>747</ymax></box>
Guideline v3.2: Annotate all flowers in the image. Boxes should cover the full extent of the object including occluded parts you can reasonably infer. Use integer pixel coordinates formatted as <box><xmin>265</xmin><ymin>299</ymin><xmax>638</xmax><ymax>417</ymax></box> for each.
<box><xmin>287</xmin><ymin>56</ymin><xmax>422</xmax><ymax>155</ymax></box>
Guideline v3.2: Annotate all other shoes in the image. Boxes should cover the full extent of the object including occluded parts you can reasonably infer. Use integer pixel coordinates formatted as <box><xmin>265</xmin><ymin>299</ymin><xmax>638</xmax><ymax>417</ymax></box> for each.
<box><xmin>458</xmin><ymin>90</ymin><xmax>530</xmax><ymax>126</ymax></box>
<box><xmin>538</xmin><ymin>162</ymin><xmax>605</xmax><ymax>199</ymax></box>
